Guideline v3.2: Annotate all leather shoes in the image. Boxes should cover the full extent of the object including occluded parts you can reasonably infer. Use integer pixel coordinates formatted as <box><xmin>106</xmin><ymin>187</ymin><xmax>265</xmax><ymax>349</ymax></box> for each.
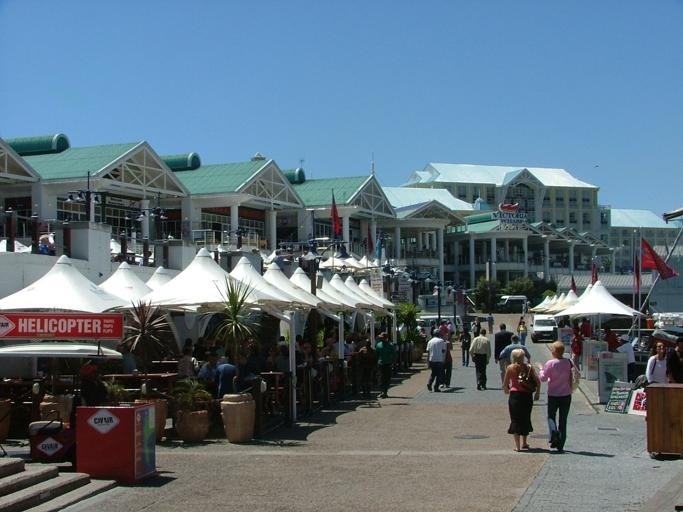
<box><xmin>427</xmin><ymin>384</ymin><xmax>432</xmax><ymax>391</ymax></box>
<box><xmin>434</xmin><ymin>389</ymin><xmax>441</xmax><ymax>392</ymax></box>
<box><xmin>551</xmin><ymin>439</ymin><xmax>559</xmax><ymax>449</ymax></box>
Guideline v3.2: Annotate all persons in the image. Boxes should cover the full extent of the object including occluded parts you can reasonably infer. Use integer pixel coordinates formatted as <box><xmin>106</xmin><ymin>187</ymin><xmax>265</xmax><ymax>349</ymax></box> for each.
<box><xmin>341</xmin><ymin>265</ymin><xmax>347</xmax><ymax>273</ymax></box>
<box><xmin>537</xmin><ymin>341</ymin><xmax>574</xmax><ymax>452</ymax></box>
<box><xmin>645</xmin><ymin>341</ymin><xmax>668</xmax><ymax>384</ymax></box>
<box><xmin>666</xmin><ymin>338</ymin><xmax>682</xmax><ymax>383</ymax></box>
<box><xmin>472</xmin><ymin>317</ymin><xmax>481</xmax><ymax>336</ymax></box>
<box><xmin>503</xmin><ymin>348</ymin><xmax>540</xmax><ymax>451</ymax></box>
<box><xmin>487</xmin><ymin>313</ymin><xmax>494</xmax><ymax>333</ymax></box>
<box><xmin>417</xmin><ymin>323</ymin><xmax>426</xmax><ymax>336</ymax></box>
<box><xmin>581</xmin><ymin>318</ymin><xmax>590</xmax><ymax>337</ymax></box>
<box><xmin>499</xmin><ymin>334</ymin><xmax>531</xmax><ymax>370</ymax></box>
<box><xmin>178</xmin><ymin>321</ymin><xmax>396</xmax><ymax>413</ymax></box>
<box><xmin>558</xmin><ymin>316</ymin><xmax>570</xmax><ymax>328</ymax></box>
<box><xmin>571</xmin><ymin>327</ymin><xmax>582</xmax><ymax>370</ymax></box>
<box><xmin>439</xmin><ymin>321</ymin><xmax>454</xmax><ymax>349</ymax></box>
<box><xmin>469</xmin><ymin>329</ymin><xmax>491</xmax><ymax>391</ymax></box>
<box><xmin>517</xmin><ymin>316</ymin><xmax>527</xmax><ymax>346</ymax></box>
<box><xmin>616</xmin><ymin>335</ymin><xmax>636</xmax><ymax>374</ymax></box>
<box><xmin>80</xmin><ymin>366</ymin><xmax>109</xmax><ymax>405</ymax></box>
<box><xmin>460</xmin><ymin>328</ymin><xmax>470</xmax><ymax>367</ymax></box>
<box><xmin>494</xmin><ymin>324</ymin><xmax>514</xmax><ymax>382</ymax></box>
<box><xmin>603</xmin><ymin>325</ymin><xmax>620</xmax><ymax>352</ymax></box>
<box><xmin>139</xmin><ymin>251</ymin><xmax>152</xmax><ymax>266</ymax></box>
<box><xmin>426</xmin><ymin>329</ymin><xmax>452</xmax><ymax>392</ymax></box>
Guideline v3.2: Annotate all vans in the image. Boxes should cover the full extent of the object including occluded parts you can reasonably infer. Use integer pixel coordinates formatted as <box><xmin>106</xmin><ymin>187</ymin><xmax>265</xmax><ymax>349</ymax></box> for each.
<box><xmin>415</xmin><ymin>315</ymin><xmax>464</xmax><ymax>339</ymax></box>
<box><xmin>496</xmin><ymin>294</ymin><xmax>527</xmax><ymax>313</ymax></box>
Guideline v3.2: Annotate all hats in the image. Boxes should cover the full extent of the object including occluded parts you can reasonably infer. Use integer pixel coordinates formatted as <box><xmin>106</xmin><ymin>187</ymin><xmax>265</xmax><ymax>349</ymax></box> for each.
<box><xmin>618</xmin><ymin>334</ymin><xmax>630</xmax><ymax>342</ymax></box>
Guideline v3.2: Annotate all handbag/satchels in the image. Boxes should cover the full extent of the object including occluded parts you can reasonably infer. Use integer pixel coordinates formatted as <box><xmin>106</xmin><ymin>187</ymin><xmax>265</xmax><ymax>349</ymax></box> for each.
<box><xmin>569</xmin><ymin>361</ymin><xmax>580</xmax><ymax>391</ymax></box>
<box><xmin>518</xmin><ymin>365</ymin><xmax>539</xmax><ymax>394</ymax></box>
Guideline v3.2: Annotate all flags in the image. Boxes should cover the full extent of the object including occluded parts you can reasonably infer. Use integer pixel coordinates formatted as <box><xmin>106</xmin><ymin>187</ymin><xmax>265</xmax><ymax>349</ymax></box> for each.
<box><xmin>641</xmin><ymin>237</ymin><xmax>680</xmax><ymax>279</ymax></box>
<box><xmin>330</xmin><ymin>193</ymin><xmax>341</xmax><ymax>235</ymax></box>
<box><xmin>368</xmin><ymin>228</ymin><xmax>373</xmax><ymax>252</ymax></box>
<box><xmin>633</xmin><ymin>255</ymin><xmax>639</xmax><ymax>293</ymax></box>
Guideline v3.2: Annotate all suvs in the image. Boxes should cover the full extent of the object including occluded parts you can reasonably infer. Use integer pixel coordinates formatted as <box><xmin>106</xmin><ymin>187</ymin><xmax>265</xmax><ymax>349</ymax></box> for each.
<box><xmin>530</xmin><ymin>314</ymin><xmax>558</xmax><ymax>343</ymax></box>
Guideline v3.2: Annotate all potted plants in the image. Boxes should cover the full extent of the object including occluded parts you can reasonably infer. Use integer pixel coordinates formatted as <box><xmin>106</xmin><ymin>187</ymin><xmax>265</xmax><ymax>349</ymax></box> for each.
<box><xmin>389</xmin><ymin>300</ymin><xmax>428</xmax><ymax>364</ymax></box>
<box><xmin>189</xmin><ymin>271</ymin><xmax>273</xmax><ymax>445</ymax></box>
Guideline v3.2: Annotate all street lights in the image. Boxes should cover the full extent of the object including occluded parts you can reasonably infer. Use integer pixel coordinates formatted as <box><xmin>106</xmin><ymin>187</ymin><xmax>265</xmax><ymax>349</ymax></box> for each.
<box><xmin>298</xmin><ymin>255</ymin><xmax>320</xmax><ymax>342</ymax></box>
<box><xmin>447</xmin><ymin>286</ymin><xmax>456</xmax><ymax>325</ymax></box>
<box><xmin>432</xmin><ymin>286</ymin><xmax>441</xmax><ymax>319</ymax></box>
<box><xmin>484</xmin><ymin>259</ymin><xmax>496</xmax><ymax>282</ymax></box>
<box><xmin>381</xmin><ymin>263</ymin><xmax>393</xmax><ymax>341</ymax></box>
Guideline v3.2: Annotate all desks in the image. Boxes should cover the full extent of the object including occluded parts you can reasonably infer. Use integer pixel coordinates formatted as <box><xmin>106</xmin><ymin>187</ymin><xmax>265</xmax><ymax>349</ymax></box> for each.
<box><xmin>0</xmin><ymin>330</ymin><xmax>413</xmax><ymax>440</ymax></box>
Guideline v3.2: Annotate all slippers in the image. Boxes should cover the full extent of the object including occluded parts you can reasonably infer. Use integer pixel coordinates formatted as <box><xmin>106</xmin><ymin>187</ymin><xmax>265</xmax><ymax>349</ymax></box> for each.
<box><xmin>522</xmin><ymin>444</ymin><xmax>529</xmax><ymax>448</ymax></box>
<box><xmin>513</xmin><ymin>447</ymin><xmax>521</xmax><ymax>452</ymax></box>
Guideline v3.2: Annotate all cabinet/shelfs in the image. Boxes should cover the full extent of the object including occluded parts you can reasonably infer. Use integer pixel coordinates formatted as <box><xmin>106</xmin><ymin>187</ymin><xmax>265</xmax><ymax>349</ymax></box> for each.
<box><xmin>640</xmin><ymin>382</ymin><xmax>683</xmax><ymax>460</ymax></box>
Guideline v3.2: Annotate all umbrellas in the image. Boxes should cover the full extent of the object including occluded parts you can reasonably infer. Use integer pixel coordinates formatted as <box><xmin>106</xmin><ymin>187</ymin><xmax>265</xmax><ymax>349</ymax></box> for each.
<box><xmin>527</xmin><ymin>281</ymin><xmax>646</xmax><ymax>351</ymax></box>
